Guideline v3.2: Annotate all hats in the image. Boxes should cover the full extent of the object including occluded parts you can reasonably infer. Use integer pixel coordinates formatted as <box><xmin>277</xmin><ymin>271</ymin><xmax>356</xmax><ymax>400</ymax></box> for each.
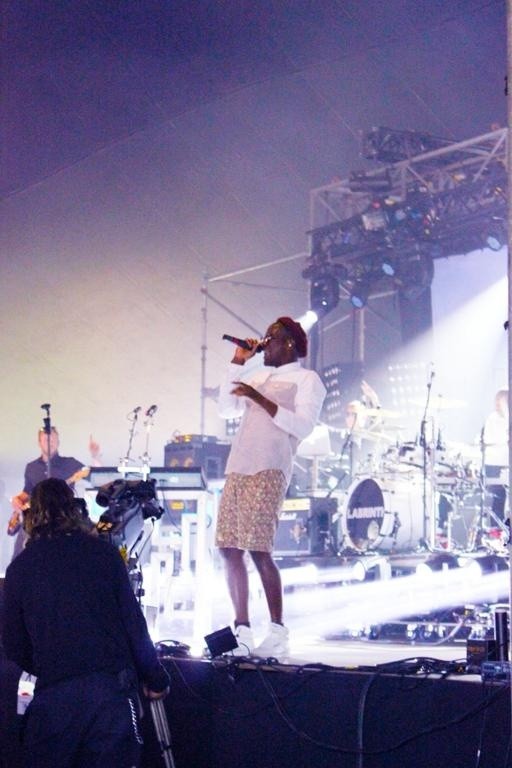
<box><xmin>347</xmin><ymin>400</ymin><xmax>367</xmax><ymax>415</ymax></box>
<box><xmin>277</xmin><ymin>317</ymin><xmax>308</xmax><ymax>357</ymax></box>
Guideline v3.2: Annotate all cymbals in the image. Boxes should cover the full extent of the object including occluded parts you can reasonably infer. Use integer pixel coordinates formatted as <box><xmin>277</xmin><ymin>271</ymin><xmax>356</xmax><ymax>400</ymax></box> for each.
<box><xmin>469</xmin><ymin>442</ymin><xmax>495</xmax><ymax>447</ymax></box>
<box><xmin>357</xmin><ymin>431</ymin><xmax>391</xmax><ymax>445</ymax></box>
<box><xmin>358</xmin><ymin>407</ymin><xmax>396</xmax><ymax>418</ymax></box>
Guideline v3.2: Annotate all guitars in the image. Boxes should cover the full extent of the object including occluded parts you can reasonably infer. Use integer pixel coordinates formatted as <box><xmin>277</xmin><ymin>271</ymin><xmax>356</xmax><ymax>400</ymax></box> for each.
<box><xmin>7</xmin><ymin>465</ymin><xmax>91</xmax><ymax>536</ymax></box>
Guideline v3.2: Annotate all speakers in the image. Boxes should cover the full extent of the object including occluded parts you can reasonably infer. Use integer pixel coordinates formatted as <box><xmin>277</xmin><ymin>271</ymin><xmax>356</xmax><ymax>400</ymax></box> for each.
<box><xmin>268</xmin><ymin>496</ymin><xmax>338</xmax><ymax>554</ymax></box>
<box><xmin>164</xmin><ymin>442</ymin><xmax>233</xmax><ymax>480</ymax></box>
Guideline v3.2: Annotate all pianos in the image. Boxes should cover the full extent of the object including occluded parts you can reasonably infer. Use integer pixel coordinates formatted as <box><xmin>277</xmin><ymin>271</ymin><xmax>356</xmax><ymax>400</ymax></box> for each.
<box><xmin>83</xmin><ymin>467</ymin><xmax>208</xmax><ymax>490</ymax></box>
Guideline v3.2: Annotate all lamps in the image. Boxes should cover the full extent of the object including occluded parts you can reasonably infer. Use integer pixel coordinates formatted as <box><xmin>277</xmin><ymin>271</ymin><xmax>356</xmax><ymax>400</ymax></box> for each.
<box><xmin>485</xmin><ymin>216</ymin><xmax>508</xmax><ymax>252</ymax></box>
<box><xmin>351</xmin><ymin>278</ymin><xmax>371</xmax><ymax>307</ymax></box>
<box><xmin>380</xmin><ymin>251</ymin><xmax>401</xmax><ymax>277</ymax></box>
<box><xmin>307</xmin><ymin>278</ymin><xmax>339</xmax><ymax>323</ymax></box>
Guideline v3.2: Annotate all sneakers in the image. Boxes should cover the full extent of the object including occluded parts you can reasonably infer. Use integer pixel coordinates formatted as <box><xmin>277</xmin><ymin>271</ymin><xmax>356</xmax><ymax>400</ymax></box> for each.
<box><xmin>221</xmin><ymin>626</ymin><xmax>255</xmax><ymax>660</ymax></box>
<box><xmin>248</xmin><ymin>621</ymin><xmax>289</xmax><ymax>663</ymax></box>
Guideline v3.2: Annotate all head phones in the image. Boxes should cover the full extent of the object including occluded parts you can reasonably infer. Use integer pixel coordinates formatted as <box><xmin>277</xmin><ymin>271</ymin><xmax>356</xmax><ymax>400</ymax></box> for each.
<box><xmin>21</xmin><ymin>479</ymin><xmax>88</xmax><ymax>531</ymax></box>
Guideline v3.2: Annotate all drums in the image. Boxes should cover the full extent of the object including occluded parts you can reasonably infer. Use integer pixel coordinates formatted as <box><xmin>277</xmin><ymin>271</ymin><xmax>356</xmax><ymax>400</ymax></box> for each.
<box><xmin>341</xmin><ymin>476</ymin><xmax>423</xmax><ymax>554</ymax></box>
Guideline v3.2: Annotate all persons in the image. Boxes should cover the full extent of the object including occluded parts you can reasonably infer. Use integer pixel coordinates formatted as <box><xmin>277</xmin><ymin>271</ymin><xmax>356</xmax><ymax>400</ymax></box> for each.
<box><xmin>212</xmin><ymin>315</ymin><xmax>329</xmax><ymax>658</ymax></box>
<box><xmin>480</xmin><ymin>392</ymin><xmax>510</xmax><ymax>528</ymax></box>
<box><xmin>0</xmin><ymin>478</ymin><xmax>171</xmax><ymax>768</ymax></box>
<box><xmin>11</xmin><ymin>425</ymin><xmax>92</xmax><ymax>564</ymax></box>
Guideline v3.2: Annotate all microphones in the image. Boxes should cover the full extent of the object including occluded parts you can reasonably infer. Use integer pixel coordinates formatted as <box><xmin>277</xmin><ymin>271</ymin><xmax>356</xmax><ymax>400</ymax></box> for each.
<box><xmin>40</xmin><ymin>403</ymin><xmax>53</xmax><ymax>434</ymax></box>
<box><xmin>132</xmin><ymin>405</ymin><xmax>141</xmax><ymax>420</ymax></box>
<box><xmin>222</xmin><ymin>333</ymin><xmax>263</xmax><ymax>353</ymax></box>
<box><xmin>425</xmin><ymin>359</ymin><xmax>434</xmax><ymax>385</ymax></box>
<box><xmin>351</xmin><ymin>393</ymin><xmax>367</xmax><ymax>415</ymax></box>
<box><xmin>145</xmin><ymin>404</ymin><xmax>160</xmax><ymax>416</ymax></box>
